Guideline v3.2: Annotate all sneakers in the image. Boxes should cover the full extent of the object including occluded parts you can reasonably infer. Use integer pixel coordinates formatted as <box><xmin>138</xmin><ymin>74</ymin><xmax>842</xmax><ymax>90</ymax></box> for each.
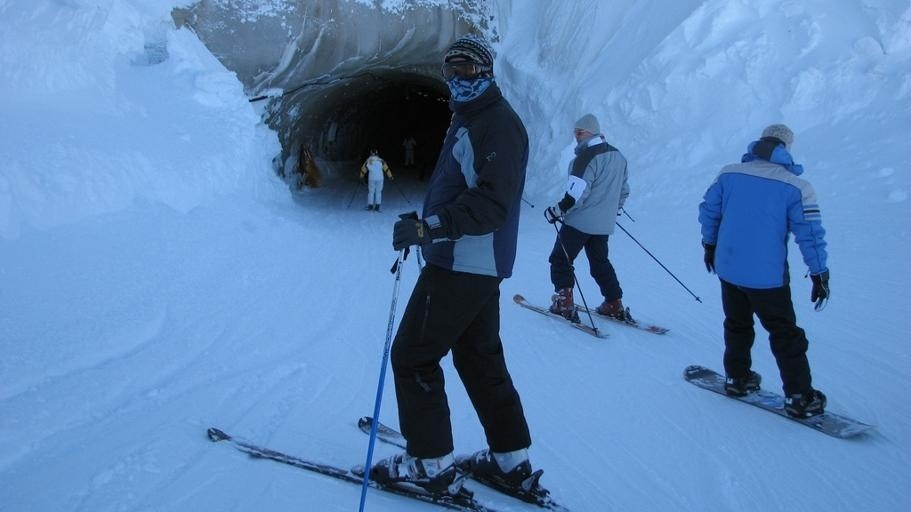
<box><xmin>595</xmin><ymin>298</ymin><xmax>625</xmax><ymax>318</ymax></box>
<box><xmin>465</xmin><ymin>444</ymin><xmax>536</xmax><ymax>491</ymax></box>
<box><xmin>366</xmin><ymin>204</ymin><xmax>380</xmax><ymax>212</ymax></box>
<box><xmin>548</xmin><ymin>289</ymin><xmax>574</xmax><ymax>316</ymax></box>
<box><xmin>371</xmin><ymin>452</ymin><xmax>457</xmax><ymax>495</ymax></box>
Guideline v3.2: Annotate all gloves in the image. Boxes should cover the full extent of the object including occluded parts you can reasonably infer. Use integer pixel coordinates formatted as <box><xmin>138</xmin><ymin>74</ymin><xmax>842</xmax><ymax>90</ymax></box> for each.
<box><xmin>783</xmin><ymin>387</ymin><xmax>828</xmax><ymax>417</ymax></box>
<box><xmin>544</xmin><ymin>206</ymin><xmax>562</xmax><ymax>224</ymax></box>
<box><xmin>392</xmin><ymin>216</ymin><xmax>433</xmax><ymax>253</ymax></box>
<box><xmin>703</xmin><ymin>244</ymin><xmax>717</xmax><ymax>274</ymax></box>
<box><xmin>809</xmin><ymin>269</ymin><xmax>831</xmax><ymax>313</ymax></box>
<box><xmin>722</xmin><ymin>368</ymin><xmax>762</xmax><ymax>394</ymax></box>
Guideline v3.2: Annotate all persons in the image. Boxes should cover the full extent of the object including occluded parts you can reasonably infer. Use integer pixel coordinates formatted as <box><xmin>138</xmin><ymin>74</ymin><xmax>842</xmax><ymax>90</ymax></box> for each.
<box><xmin>545</xmin><ymin>114</ymin><xmax>630</xmax><ymax>316</ymax></box>
<box><xmin>403</xmin><ymin>135</ymin><xmax>416</xmax><ymax>165</ymax></box>
<box><xmin>699</xmin><ymin>124</ymin><xmax>830</xmax><ymax>418</ymax></box>
<box><xmin>370</xmin><ymin>33</ymin><xmax>531</xmax><ymax>495</ymax></box>
<box><xmin>360</xmin><ymin>147</ymin><xmax>394</xmax><ymax>211</ymax></box>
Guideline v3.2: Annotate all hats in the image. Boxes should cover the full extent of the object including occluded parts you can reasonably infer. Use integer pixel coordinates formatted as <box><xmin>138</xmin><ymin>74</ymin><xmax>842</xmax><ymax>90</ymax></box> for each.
<box><xmin>572</xmin><ymin>113</ymin><xmax>600</xmax><ymax>134</ymax></box>
<box><xmin>370</xmin><ymin>148</ymin><xmax>378</xmax><ymax>154</ymax></box>
<box><xmin>443</xmin><ymin>33</ymin><xmax>495</xmax><ymax>64</ymax></box>
<box><xmin>760</xmin><ymin>117</ymin><xmax>796</xmax><ymax>147</ymax></box>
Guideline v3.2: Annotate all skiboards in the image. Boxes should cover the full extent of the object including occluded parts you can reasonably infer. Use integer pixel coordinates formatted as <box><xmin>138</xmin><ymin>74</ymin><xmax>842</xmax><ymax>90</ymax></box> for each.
<box><xmin>513</xmin><ymin>294</ymin><xmax>671</xmax><ymax>338</ymax></box>
<box><xmin>208</xmin><ymin>417</ymin><xmax>570</xmax><ymax>511</ymax></box>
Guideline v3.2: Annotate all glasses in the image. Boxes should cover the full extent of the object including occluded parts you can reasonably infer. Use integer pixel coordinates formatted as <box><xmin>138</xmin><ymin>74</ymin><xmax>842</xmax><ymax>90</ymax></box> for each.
<box><xmin>441</xmin><ymin>65</ymin><xmax>493</xmax><ymax>79</ymax></box>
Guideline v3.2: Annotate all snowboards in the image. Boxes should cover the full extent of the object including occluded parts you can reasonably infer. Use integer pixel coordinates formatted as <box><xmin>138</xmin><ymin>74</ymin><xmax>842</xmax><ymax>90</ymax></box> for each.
<box><xmin>685</xmin><ymin>364</ymin><xmax>876</xmax><ymax>440</ymax></box>
